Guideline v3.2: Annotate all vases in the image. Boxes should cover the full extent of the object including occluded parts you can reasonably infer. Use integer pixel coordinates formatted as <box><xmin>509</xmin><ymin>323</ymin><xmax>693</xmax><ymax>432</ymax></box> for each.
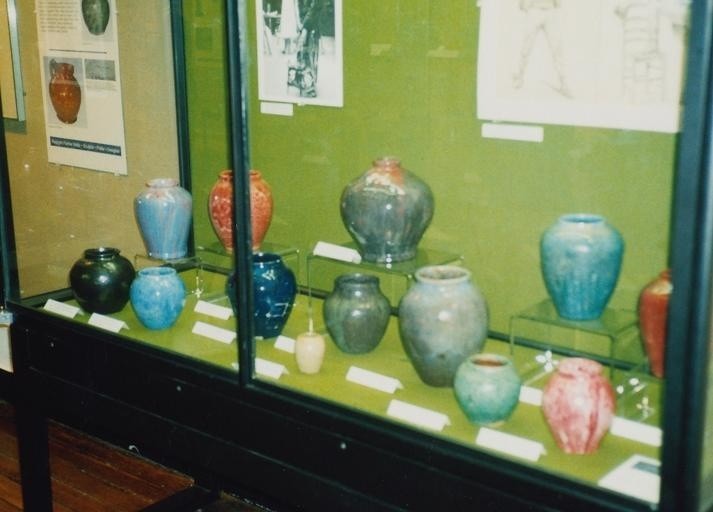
<box><xmin>207</xmin><ymin>170</ymin><xmax>274</xmax><ymax>254</ymax></box>
<box><xmin>134</xmin><ymin>178</ymin><xmax>191</xmax><ymax>260</ymax></box>
<box><xmin>637</xmin><ymin>270</ymin><xmax>673</xmax><ymax>379</ymax></box>
<box><xmin>323</xmin><ymin>273</ymin><xmax>391</xmax><ymax>353</ymax></box>
<box><xmin>338</xmin><ymin>158</ymin><xmax>435</xmax><ymax>263</ymax></box>
<box><xmin>70</xmin><ymin>248</ymin><xmax>136</xmax><ymax>316</ymax></box>
<box><xmin>398</xmin><ymin>266</ymin><xmax>492</xmax><ymax>390</ymax></box>
<box><xmin>129</xmin><ymin>268</ymin><xmax>187</xmax><ymax>329</ymax></box>
<box><xmin>44</xmin><ymin>59</ymin><xmax>82</xmax><ymax>124</ymax></box>
<box><xmin>80</xmin><ymin>0</ymin><xmax>111</xmax><ymax>34</ymax></box>
<box><xmin>541</xmin><ymin>214</ymin><xmax>625</xmax><ymax>322</ymax></box>
<box><xmin>454</xmin><ymin>354</ymin><xmax>521</xmax><ymax>426</ymax></box>
<box><xmin>224</xmin><ymin>255</ymin><xmax>296</xmax><ymax>340</ymax></box>
<box><xmin>538</xmin><ymin>360</ymin><xmax>616</xmax><ymax>459</ymax></box>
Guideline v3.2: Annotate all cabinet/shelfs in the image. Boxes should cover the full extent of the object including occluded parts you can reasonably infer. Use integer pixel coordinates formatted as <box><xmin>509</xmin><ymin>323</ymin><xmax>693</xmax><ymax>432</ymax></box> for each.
<box><xmin>0</xmin><ymin>1</ymin><xmax>710</xmax><ymax>510</ymax></box>
